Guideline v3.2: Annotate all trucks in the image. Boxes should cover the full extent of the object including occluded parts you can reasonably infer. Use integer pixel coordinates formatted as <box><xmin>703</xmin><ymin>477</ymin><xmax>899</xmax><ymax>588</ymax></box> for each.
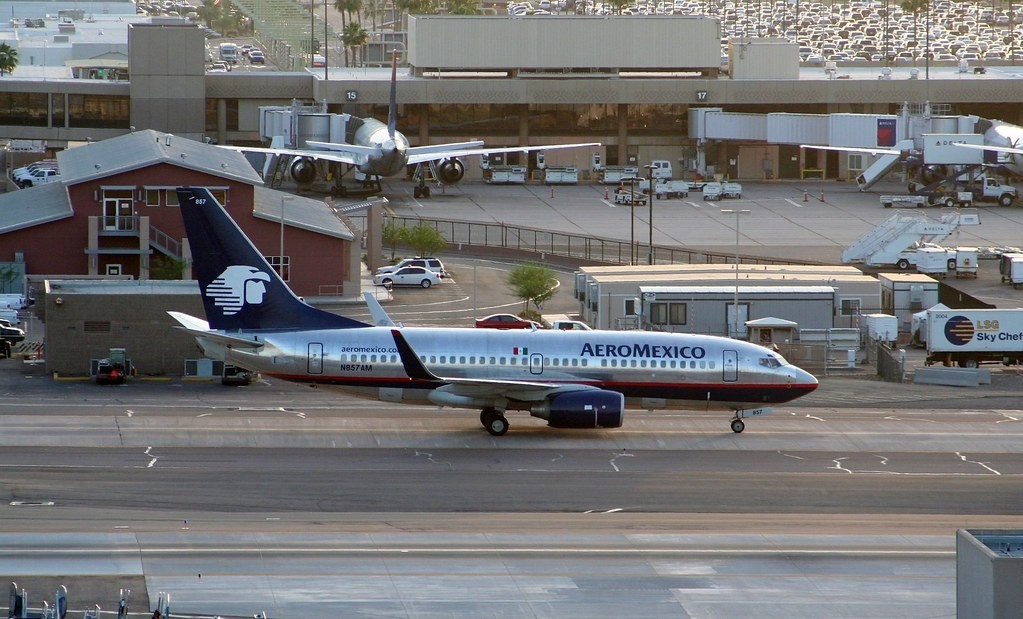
<box><xmin>840</xmin><ymin>210</ymin><xmax>960</xmax><ymax>269</ymax></box>
<box><xmin>916</xmin><ymin>133</ymin><xmax>1018</xmax><ymax>208</ymax></box>
<box><xmin>926</xmin><ymin>309</ymin><xmax>1023</xmax><ymax>368</ymax></box>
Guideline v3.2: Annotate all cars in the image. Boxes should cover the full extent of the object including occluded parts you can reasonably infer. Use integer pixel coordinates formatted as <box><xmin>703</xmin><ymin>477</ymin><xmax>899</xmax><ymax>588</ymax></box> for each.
<box><xmin>476</xmin><ymin>314</ymin><xmax>544</xmax><ymax>329</ymax></box>
<box><xmin>96</xmin><ymin>360</ymin><xmax>126</xmax><ymax>385</ymax></box>
<box><xmin>0</xmin><ymin>293</ymin><xmax>26</xmax><ymax>346</ymax></box>
<box><xmin>11</xmin><ymin>161</ymin><xmax>61</xmax><ymax>189</ymax></box>
<box><xmin>508</xmin><ymin>0</ymin><xmax>1023</xmax><ymax>62</ymax></box>
<box><xmin>373</xmin><ymin>266</ymin><xmax>442</xmax><ymax>288</ymax></box>
<box><xmin>133</xmin><ymin>0</ymin><xmax>265</xmax><ymax>71</ymax></box>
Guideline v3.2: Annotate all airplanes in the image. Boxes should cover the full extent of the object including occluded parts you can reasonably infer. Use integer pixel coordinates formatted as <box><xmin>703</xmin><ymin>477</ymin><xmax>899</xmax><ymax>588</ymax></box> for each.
<box><xmin>217</xmin><ymin>47</ymin><xmax>602</xmax><ymax>198</ymax></box>
<box><xmin>166</xmin><ymin>185</ymin><xmax>819</xmax><ymax>436</ymax></box>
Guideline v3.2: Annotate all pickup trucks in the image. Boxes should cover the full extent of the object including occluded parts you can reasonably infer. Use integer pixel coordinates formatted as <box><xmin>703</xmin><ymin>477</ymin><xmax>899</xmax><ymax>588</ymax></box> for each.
<box><xmin>554</xmin><ymin>321</ymin><xmax>592</xmax><ymax>330</ymax></box>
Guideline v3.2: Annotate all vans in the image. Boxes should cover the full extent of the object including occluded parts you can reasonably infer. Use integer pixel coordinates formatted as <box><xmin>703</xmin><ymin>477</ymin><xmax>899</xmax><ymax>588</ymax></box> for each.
<box><xmin>220</xmin><ymin>43</ymin><xmax>237</xmax><ymax>64</ymax></box>
<box><xmin>647</xmin><ymin>160</ymin><xmax>672</xmax><ymax>180</ymax></box>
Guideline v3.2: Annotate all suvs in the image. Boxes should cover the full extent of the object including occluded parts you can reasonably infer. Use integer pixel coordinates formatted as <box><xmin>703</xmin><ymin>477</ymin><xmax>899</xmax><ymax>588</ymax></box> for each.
<box><xmin>376</xmin><ymin>257</ymin><xmax>444</xmax><ymax>278</ymax></box>
